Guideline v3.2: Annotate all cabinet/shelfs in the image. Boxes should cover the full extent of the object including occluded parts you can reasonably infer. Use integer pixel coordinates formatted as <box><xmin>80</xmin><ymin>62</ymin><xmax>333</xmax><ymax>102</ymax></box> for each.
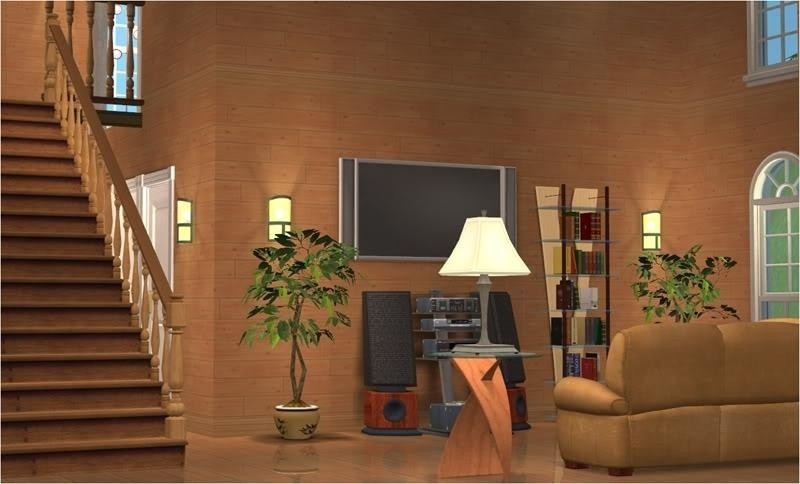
<box><xmin>535</xmin><ymin>184</ymin><xmax>621</xmax><ymax>384</ymax></box>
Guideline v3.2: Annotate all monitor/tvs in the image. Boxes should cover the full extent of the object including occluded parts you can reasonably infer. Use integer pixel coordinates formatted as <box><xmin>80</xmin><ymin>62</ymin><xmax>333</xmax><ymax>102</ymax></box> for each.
<box><xmin>360</xmin><ymin>160</ymin><xmax>504</xmax><ymax>262</ymax></box>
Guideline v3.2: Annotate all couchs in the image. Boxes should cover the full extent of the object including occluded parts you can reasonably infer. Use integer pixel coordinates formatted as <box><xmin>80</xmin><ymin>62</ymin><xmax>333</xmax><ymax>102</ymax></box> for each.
<box><xmin>553</xmin><ymin>317</ymin><xmax>800</xmax><ymax>476</ymax></box>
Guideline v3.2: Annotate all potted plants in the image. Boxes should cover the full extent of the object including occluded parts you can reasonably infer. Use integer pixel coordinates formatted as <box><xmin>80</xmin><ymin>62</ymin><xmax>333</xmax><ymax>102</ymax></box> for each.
<box><xmin>237</xmin><ymin>229</ymin><xmax>368</xmax><ymax>440</ymax></box>
<box><xmin>427</xmin><ymin>351</ymin><xmax>542</xmax><ymax>479</ymax></box>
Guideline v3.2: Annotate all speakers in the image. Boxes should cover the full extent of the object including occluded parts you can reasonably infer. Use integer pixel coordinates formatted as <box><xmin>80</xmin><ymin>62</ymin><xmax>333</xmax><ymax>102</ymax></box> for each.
<box><xmin>505</xmin><ymin>167</ymin><xmax>515</xmax><ymax>245</ymax></box>
<box><xmin>470</xmin><ymin>291</ymin><xmax>531</xmax><ymax>431</ymax></box>
<box><xmin>338</xmin><ymin>156</ymin><xmax>356</xmax><ymax>259</ymax></box>
<box><xmin>361</xmin><ymin>289</ymin><xmax>421</xmax><ymax>437</ymax></box>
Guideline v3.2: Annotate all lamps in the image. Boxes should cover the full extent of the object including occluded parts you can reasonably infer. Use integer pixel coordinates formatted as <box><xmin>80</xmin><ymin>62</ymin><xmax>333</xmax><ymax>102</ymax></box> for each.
<box><xmin>438</xmin><ymin>208</ymin><xmax>532</xmax><ymax>353</ymax></box>
<box><xmin>176</xmin><ymin>197</ymin><xmax>194</xmax><ymax>244</ymax></box>
<box><xmin>267</xmin><ymin>195</ymin><xmax>292</xmax><ymax>242</ymax></box>
<box><xmin>641</xmin><ymin>210</ymin><xmax>662</xmax><ymax>251</ymax></box>
